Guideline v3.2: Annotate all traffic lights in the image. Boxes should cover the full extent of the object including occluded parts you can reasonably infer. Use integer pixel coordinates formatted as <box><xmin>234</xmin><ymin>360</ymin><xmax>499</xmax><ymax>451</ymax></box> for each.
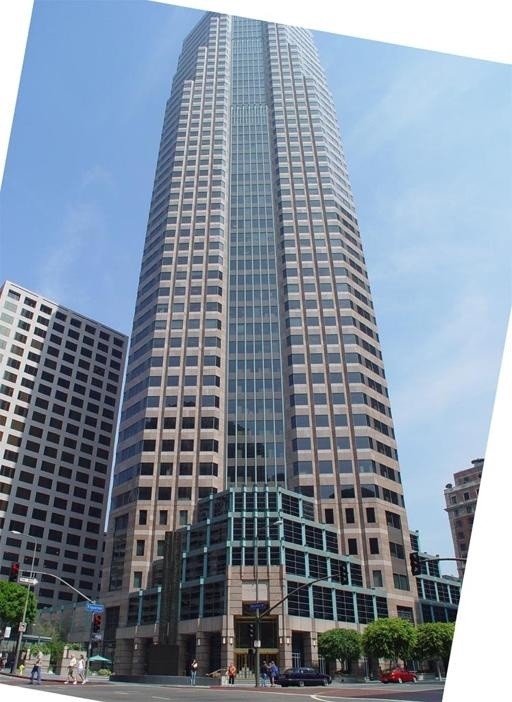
<box><xmin>409</xmin><ymin>552</ymin><xmax>421</xmax><ymax>576</ymax></box>
<box><xmin>339</xmin><ymin>560</ymin><xmax>349</xmax><ymax>586</ymax></box>
<box><xmin>8</xmin><ymin>562</ymin><xmax>20</xmax><ymax>582</ymax></box>
<box><xmin>247</xmin><ymin>623</ymin><xmax>256</xmax><ymax>640</ymax></box>
<box><xmin>93</xmin><ymin>613</ymin><xmax>102</xmax><ymax>633</ymax></box>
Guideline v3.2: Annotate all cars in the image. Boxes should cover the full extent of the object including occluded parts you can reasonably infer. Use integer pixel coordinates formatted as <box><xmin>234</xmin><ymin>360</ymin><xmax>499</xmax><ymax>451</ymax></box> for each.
<box><xmin>378</xmin><ymin>666</ymin><xmax>418</xmax><ymax>684</ymax></box>
<box><xmin>270</xmin><ymin>666</ymin><xmax>332</xmax><ymax>688</ymax></box>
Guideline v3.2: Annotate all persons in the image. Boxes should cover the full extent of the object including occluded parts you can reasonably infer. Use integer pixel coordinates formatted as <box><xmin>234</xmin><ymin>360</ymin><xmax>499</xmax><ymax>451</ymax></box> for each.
<box><xmin>28</xmin><ymin>648</ymin><xmax>43</xmax><ymax>685</ymax></box>
<box><xmin>268</xmin><ymin>660</ymin><xmax>278</xmax><ymax>687</ymax></box>
<box><xmin>261</xmin><ymin>659</ymin><xmax>270</xmax><ymax>686</ymax></box>
<box><xmin>228</xmin><ymin>663</ymin><xmax>236</xmax><ymax>684</ymax></box>
<box><xmin>191</xmin><ymin>658</ymin><xmax>198</xmax><ymax>685</ymax></box>
<box><xmin>73</xmin><ymin>654</ymin><xmax>86</xmax><ymax>684</ymax></box>
<box><xmin>64</xmin><ymin>653</ymin><xmax>78</xmax><ymax>684</ymax></box>
